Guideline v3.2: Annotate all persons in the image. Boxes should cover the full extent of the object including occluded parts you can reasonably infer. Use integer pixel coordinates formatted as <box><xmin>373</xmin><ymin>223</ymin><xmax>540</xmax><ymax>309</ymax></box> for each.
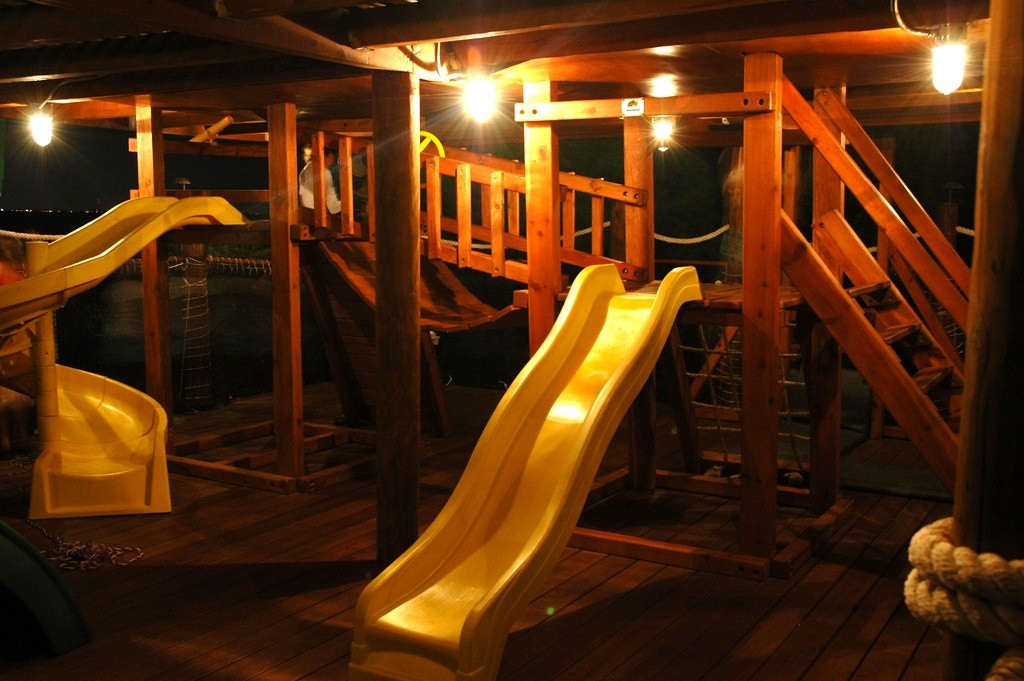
<box><xmin>297</xmin><ymin>147</ymin><xmax>344</xmax><ymax>215</ymax></box>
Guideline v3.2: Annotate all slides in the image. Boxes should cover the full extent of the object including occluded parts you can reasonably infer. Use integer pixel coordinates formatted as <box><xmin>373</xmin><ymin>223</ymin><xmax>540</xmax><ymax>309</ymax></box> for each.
<box><xmin>348</xmin><ymin>263</ymin><xmax>703</xmax><ymax>680</ymax></box>
<box><xmin>1</xmin><ymin>195</ymin><xmax>248</xmax><ymax>521</ymax></box>
